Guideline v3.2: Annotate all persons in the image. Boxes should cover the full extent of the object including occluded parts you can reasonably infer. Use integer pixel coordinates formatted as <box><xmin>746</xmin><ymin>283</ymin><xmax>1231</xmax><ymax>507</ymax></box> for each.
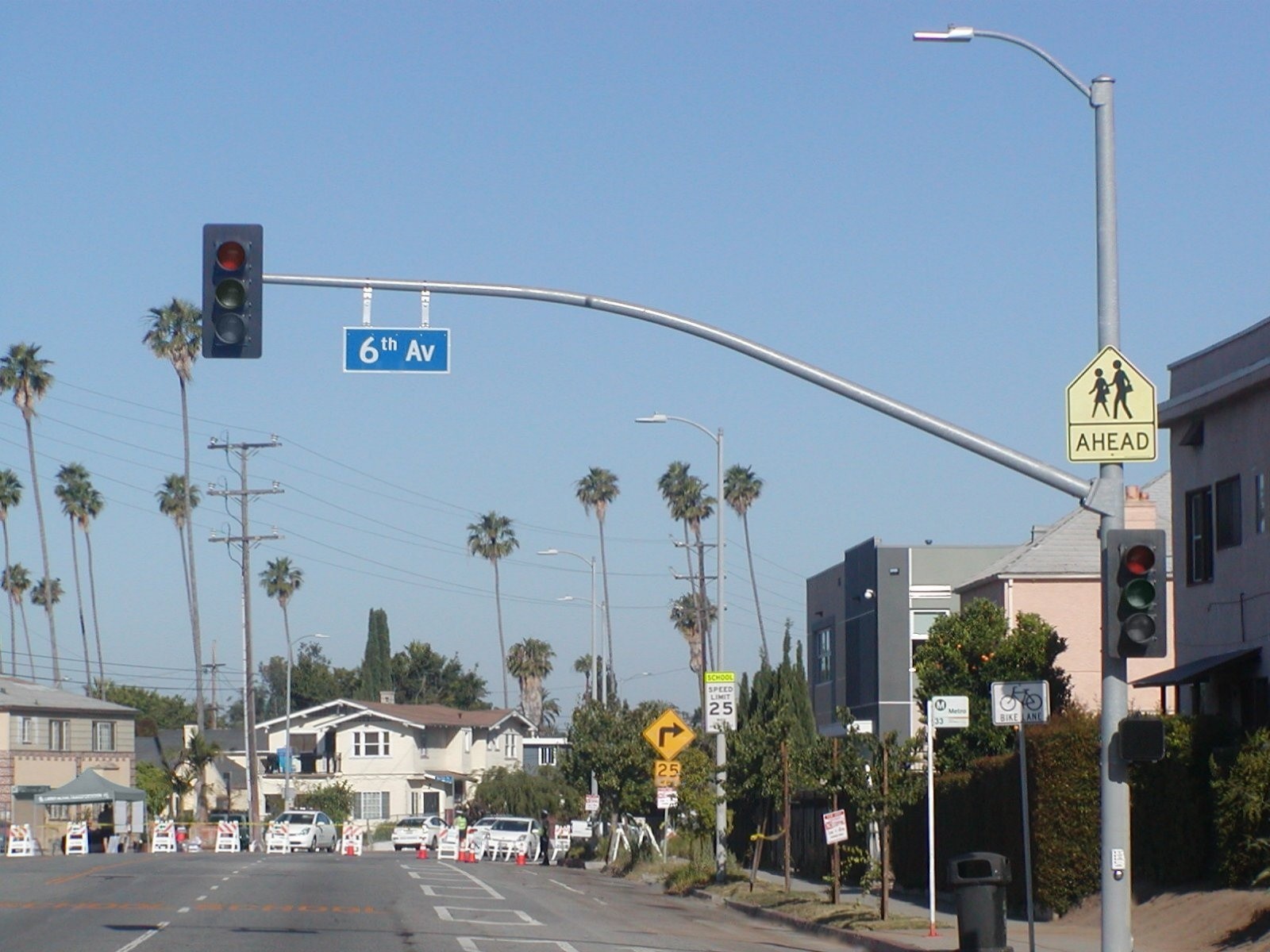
<box><xmin>98</xmin><ymin>804</ymin><xmax>113</xmax><ymax>852</ymax></box>
<box><xmin>532</xmin><ymin>809</ymin><xmax>551</xmax><ymax>866</ymax></box>
<box><xmin>452</xmin><ymin>810</ymin><xmax>468</xmax><ymax>845</ymax></box>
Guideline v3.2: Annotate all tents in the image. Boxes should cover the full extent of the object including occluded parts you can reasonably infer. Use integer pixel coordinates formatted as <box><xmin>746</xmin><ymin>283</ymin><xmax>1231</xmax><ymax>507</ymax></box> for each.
<box><xmin>33</xmin><ymin>769</ymin><xmax>151</xmax><ymax>854</ymax></box>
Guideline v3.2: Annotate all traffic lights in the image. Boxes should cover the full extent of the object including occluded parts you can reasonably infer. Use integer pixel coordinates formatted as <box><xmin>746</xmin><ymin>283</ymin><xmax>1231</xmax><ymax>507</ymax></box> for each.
<box><xmin>201</xmin><ymin>222</ymin><xmax>264</xmax><ymax>358</ymax></box>
<box><xmin>1105</xmin><ymin>528</ymin><xmax>1167</xmax><ymax>657</ymax></box>
<box><xmin>1119</xmin><ymin>718</ymin><xmax>1166</xmax><ymax>762</ymax></box>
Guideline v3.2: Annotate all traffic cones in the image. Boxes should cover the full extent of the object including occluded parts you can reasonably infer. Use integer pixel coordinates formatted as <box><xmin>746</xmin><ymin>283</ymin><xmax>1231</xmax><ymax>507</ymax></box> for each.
<box><xmin>515</xmin><ymin>843</ymin><xmax>528</xmax><ymax>865</ymax></box>
<box><xmin>416</xmin><ymin>838</ymin><xmax>430</xmax><ymax>859</ymax></box>
<box><xmin>455</xmin><ymin>837</ymin><xmax>467</xmax><ymax>862</ymax></box>
<box><xmin>464</xmin><ymin>839</ymin><xmax>479</xmax><ymax>863</ymax></box>
<box><xmin>343</xmin><ymin>834</ymin><xmax>357</xmax><ymax>856</ymax></box>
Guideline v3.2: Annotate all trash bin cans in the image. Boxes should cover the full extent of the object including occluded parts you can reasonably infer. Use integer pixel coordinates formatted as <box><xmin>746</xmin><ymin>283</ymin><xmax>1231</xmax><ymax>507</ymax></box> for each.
<box><xmin>947</xmin><ymin>852</ymin><xmax>1012</xmax><ymax>952</ymax></box>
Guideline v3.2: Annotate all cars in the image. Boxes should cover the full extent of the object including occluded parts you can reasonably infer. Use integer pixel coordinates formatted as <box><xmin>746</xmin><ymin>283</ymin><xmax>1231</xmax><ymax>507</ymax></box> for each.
<box><xmin>390</xmin><ymin>814</ymin><xmax>450</xmax><ymax>852</ymax></box>
<box><xmin>439</xmin><ymin>815</ymin><xmax>541</xmax><ymax>858</ymax></box>
<box><xmin>264</xmin><ymin>810</ymin><xmax>338</xmax><ymax>854</ymax></box>
<box><xmin>209</xmin><ymin>813</ymin><xmax>249</xmax><ymax>852</ymax></box>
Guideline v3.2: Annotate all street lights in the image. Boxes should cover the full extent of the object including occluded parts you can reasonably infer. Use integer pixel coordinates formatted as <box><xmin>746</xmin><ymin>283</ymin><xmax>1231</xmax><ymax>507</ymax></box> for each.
<box><xmin>634</xmin><ymin>412</ymin><xmax>728</xmax><ymax>884</ymax></box>
<box><xmin>557</xmin><ymin>596</ymin><xmax>607</xmax><ymax>711</ymax></box>
<box><xmin>283</xmin><ymin>634</ymin><xmax>330</xmax><ymax>813</ymax></box>
<box><xmin>913</xmin><ymin>25</ymin><xmax>1134</xmax><ymax>952</ymax></box>
<box><xmin>536</xmin><ymin>547</ymin><xmax>599</xmax><ymax>825</ymax></box>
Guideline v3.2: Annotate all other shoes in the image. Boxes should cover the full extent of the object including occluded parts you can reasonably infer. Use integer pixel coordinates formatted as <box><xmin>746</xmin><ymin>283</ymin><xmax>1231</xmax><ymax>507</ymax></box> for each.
<box><xmin>538</xmin><ymin>856</ymin><xmax>550</xmax><ymax>866</ymax></box>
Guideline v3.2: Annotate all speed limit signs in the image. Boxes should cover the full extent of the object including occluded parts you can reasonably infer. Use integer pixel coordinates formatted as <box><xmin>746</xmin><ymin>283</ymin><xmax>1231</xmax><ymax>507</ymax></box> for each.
<box><xmin>655</xmin><ymin>761</ymin><xmax>681</xmax><ymax>788</ymax></box>
<box><xmin>706</xmin><ymin>673</ymin><xmax>737</xmax><ymax>734</ymax></box>
